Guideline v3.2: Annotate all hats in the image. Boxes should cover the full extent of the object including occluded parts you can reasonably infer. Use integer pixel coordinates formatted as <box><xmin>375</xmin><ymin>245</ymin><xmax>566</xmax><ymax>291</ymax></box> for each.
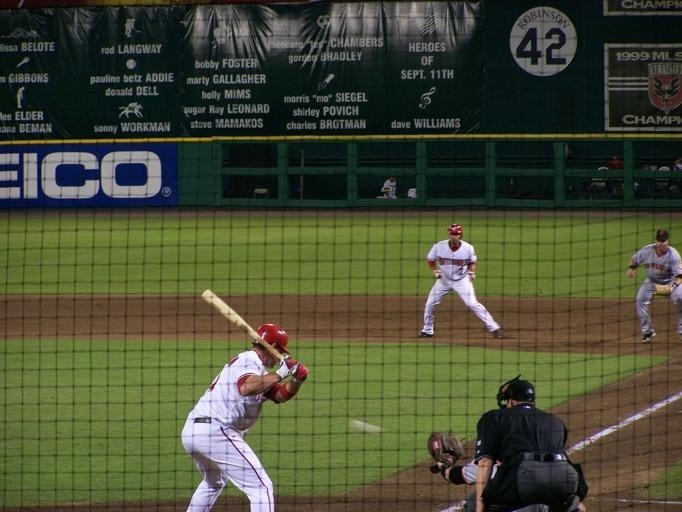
<box><xmin>655</xmin><ymin>229</ymin><xmax>668</xmax><ymax>242</ymax></box>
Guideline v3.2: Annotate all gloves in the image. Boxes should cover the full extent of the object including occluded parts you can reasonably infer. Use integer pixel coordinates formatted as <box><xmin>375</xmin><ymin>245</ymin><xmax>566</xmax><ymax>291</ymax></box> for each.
<box><xmin>275</xmin><ymin>356</ymin><xmax>309</xmax><ymax>384</ymax></box>
<box><xmin>433</xmin><ymin>269</ymin><xmax>443</xmax><ymax>280</ymax></box>
<box><xmin>468</xmin><ymin>271</ymin><xmax>476</xmax><ymax>281</ymax></box>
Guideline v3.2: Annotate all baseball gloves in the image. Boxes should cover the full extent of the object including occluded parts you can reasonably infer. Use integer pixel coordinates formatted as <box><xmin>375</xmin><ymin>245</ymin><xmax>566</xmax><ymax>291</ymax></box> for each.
<box><xmin>653</xmin><ymin>285</ymin><xmax>673</xmax><ymax>296</ymax></box>
<box><xmin>428</xmin><ymin>431</ymin><xmax>463</xmax><ymax>473</ymax></box>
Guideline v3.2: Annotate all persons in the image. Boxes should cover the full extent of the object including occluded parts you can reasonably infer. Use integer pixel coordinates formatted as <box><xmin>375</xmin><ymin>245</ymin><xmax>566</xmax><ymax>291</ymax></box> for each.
<box><xmin>626</xmin><ymin>229</ymin><xmax>682</xmax><ymax>341</ymax></box>
<box><xmin>407</xmin><ymin>183</ymin><xmax>417</xmax><ymax>199</ymax></box>
<box><xmin>466</xmin><ymin>377</ymin><xmax>579</xmax><ymax>512</ymax></box>
<box><xmin>436</xmin><ymin>444</ymin><xmax>587</xmax><ymax>512</ymax></box>
<box><xmin>417</xmin><ymin>222</ymin><xmax>504</xmax><ymax>340</ymax></box>
<box><xmin>586</xmin><ymin>155</ymin><xmax>681</xmax><ymax>199</ymax></box>
<box><xmin>180</xmin><ymin>323</ymin><xmax>308</xmax><ymax>512</ymax></box>
<box><xmin>377</xmin><ymin>176</ymin><xmax>399</xmax><ymax>201</ymax></box>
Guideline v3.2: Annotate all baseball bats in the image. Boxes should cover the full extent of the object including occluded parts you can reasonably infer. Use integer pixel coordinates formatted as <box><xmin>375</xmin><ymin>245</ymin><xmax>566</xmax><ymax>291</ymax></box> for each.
<box><xmin>201</xmin><ymin>289</ymin><xmax>283</xmax><ymax>359</ymax></box>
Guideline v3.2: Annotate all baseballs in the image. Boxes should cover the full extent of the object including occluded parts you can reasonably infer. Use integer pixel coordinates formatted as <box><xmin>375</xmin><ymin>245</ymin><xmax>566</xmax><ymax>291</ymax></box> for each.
<box><xmin>126</xmin><ymin>60</ymin><xmax>135</xmax><ymax>69</ymax></box>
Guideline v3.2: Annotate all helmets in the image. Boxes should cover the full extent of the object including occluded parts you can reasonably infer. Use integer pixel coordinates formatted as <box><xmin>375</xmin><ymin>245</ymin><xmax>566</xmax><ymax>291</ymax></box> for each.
<box><xmin>448</xmin><ymin>223</ymin><xmax>463</xmax><ymax>237</ymax></box>
<box><xmin>251</xmin><ymin>323</ymin><xmax>290</xmax><ymax>356</ymax></box>
<box><xmin>504</xmin><ymin>380</ymin><xmax>536</xmax><ymax>403</ymax></box>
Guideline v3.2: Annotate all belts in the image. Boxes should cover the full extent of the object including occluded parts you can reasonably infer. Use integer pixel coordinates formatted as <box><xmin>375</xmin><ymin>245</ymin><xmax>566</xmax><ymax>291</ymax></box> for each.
<box><xmin>513</xmin><ymin>451</ymin><xmax>568</xmax><ymax>462</ymax></box>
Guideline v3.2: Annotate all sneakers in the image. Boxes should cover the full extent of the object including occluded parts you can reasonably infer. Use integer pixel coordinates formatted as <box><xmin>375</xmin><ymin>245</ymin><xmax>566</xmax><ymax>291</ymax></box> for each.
<box><xmin>640</xmin><ymin>329</ymin><xmax>656</xmax><ymax>342</ymax></box>
<box><xmin>493</xmin><ymin>328</ymin><xmax>506</xmax><ymax>339</ymax></box>
<box><xmin>417</xmin><ymin>332</ymin><xmax>433</xmax><ymax>338</ymax></box>
